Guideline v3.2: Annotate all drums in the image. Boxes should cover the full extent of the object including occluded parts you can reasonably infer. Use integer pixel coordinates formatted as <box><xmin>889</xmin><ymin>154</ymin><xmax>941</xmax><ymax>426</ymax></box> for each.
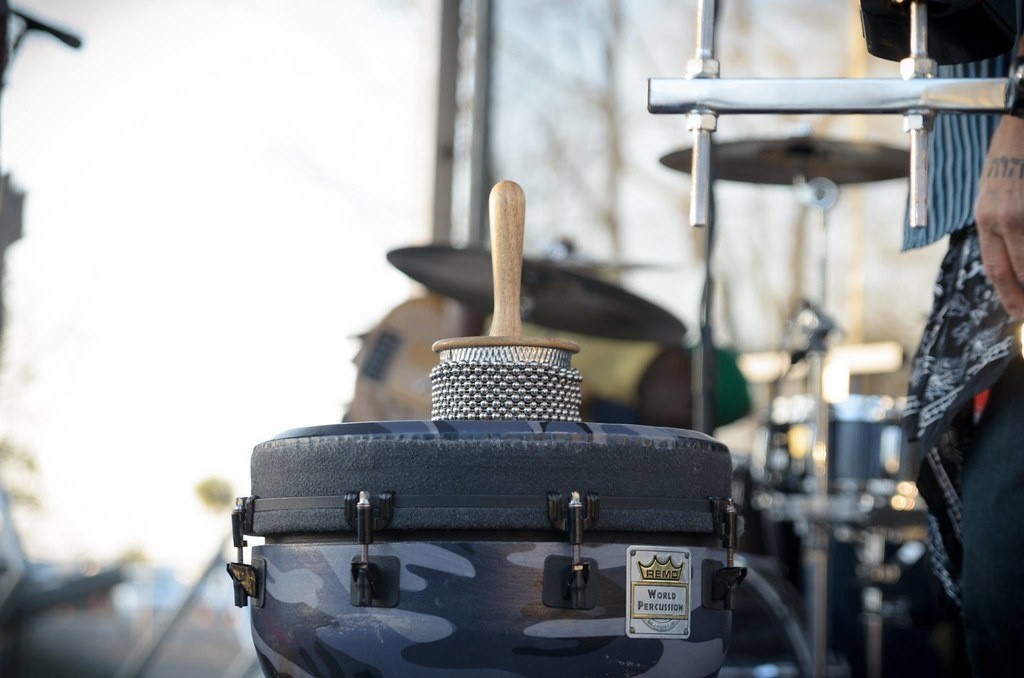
<box><xmin>761</xmin><ymin>392</ymin><xmax>903</xmax><ymax>495</ymax></box>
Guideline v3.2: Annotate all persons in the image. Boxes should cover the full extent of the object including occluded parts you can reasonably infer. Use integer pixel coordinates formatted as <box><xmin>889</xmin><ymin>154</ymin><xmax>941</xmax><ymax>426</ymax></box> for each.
<box><xmin>860</xmin><ymin>0</ymin><xmax>1023</xmax><ymax>677</ymax></box>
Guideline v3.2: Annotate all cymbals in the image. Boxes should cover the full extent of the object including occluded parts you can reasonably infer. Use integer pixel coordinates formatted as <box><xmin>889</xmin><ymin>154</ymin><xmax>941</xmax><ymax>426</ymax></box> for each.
<box><xmin>382</xmin><ymin>243</ymin><xmax>687</xmax><ymax>339</ymax></box>
<box><xmin>659</xmin><ymin>135</ymin><xmax>908</xmax><ymax>184</ymax></box>
